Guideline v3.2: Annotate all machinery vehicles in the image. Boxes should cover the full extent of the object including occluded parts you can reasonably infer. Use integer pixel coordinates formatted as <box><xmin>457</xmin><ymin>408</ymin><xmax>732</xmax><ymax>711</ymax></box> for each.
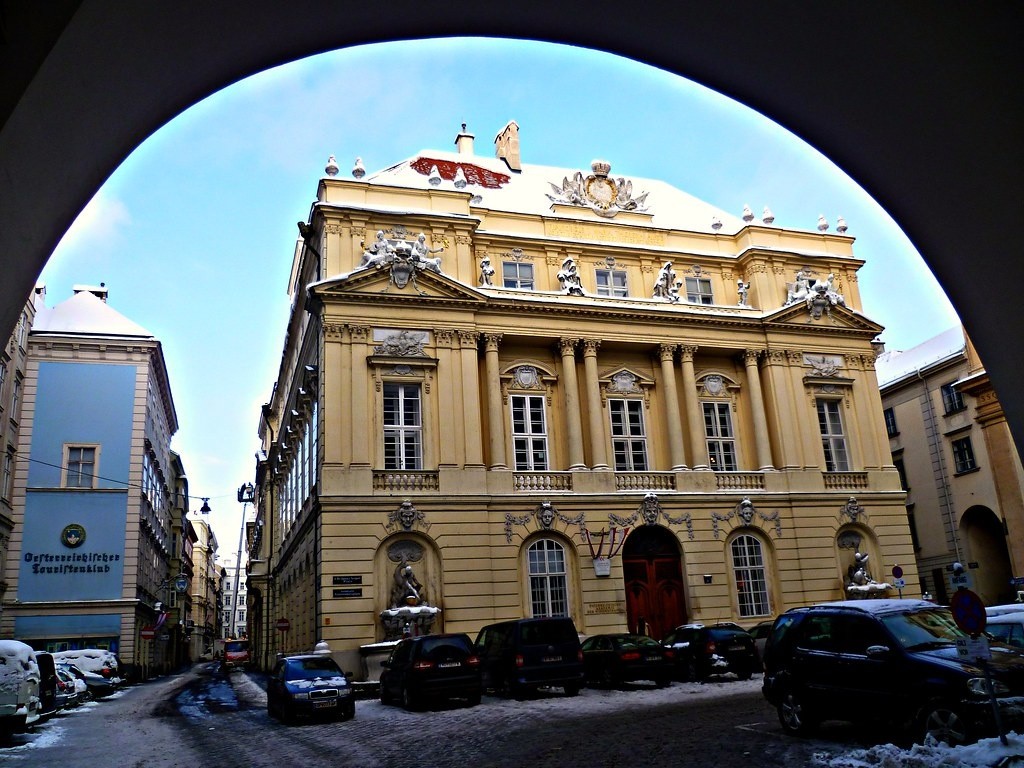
<box><xmin>199</xmin><ymin>487</ymin><xmax>256</xmax><ymax>662</ymax></box>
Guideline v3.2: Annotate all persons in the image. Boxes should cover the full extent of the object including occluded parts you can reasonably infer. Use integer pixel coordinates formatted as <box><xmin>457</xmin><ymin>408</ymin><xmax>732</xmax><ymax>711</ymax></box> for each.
<box><xmin>847</xmin><ymin>552</ymin><xmax>872</xmax><ymax>586</ymax></box>
<box><xmin>359</xmin><ymin>230</ymin><xmax>388</xmax><ymax>269</ymax></box>
<box><xmin>403</xmin><ymin>622</ymin><xmax>411</xmax><ymax>640</ymax></box>
<box><xmin>411</xmin><ymin>232</ymin><xmax>444</xmax><ymax>274</ymax></box>
<box><xmin>401</xmin><ymin>565</ymin><xmax>424</xmax><ymax>606</ymax></box>
<box><xmin>475</xmin><ymin>256</ymin><xmax>845</xmax><ymax>323</ymax></box>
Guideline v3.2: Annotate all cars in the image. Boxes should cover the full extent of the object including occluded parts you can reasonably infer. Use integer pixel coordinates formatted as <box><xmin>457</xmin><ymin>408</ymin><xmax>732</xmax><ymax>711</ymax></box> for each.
<box><xmin>746</xmin><ymin>620</ymin><xmax>777</xmax><ymax>671</ymax></box>
<box><xmin>982</xmin><ymin>603</ymin><xmax>1024</xmax><ymax>650</ymax></box>
<box><xmin>267</xmin><ymin>654</ymin><xmax>355</xmax><ymax>726</ymax></box>
<box><xmin>580</xmin><ymin>633</ymin><xmax>679</xmax><ymax>691</ymax></box>
<box><xmin>54</xmin><ymin>664</ymin><xmax>115</xmax><ymax>714</ymax></box>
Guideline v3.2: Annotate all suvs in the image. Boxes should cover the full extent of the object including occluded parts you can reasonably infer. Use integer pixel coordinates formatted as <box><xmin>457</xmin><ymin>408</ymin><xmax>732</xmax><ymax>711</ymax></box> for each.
<box><xmin>52</xmin><ymin>649</ymin><xmax>127</xmax><ymax>692</ymax></box>
<box><xmin>661</xmin><ymin>621</ymin><xmax>756</xmax><ymax>685</ymax></box>
<box><xmin>380</xmin><ymin>633</ymin><xmax>481</xmax><ymax>711</ymax></box>
<box><xmin>762</xmin><ymin>598</ymin><xmax>1024</xmax><ymax>748</ymax></box>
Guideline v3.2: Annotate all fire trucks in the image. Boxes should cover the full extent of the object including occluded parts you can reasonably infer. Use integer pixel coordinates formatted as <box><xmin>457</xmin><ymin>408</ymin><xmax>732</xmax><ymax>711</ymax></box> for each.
<box><xmin>224</xmin><ymin>634</ymin><xmax>253</xmax><ymax>671</ymax></box>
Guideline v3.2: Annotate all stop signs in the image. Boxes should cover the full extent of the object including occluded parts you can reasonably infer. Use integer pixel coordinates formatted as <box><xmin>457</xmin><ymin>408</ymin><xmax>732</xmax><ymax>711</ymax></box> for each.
<box><xmin>277</xmin><ymin>618</ymin><xmax>290</xmax><ymax>632</ymax></box>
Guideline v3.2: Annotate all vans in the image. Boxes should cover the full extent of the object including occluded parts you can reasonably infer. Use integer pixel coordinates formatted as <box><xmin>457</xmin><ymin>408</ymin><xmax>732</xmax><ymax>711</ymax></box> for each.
<box><xmin>474</xmin><ymin>616</ymin><xmax>585</xmax><ymax>700</ymax></box>
<box><xmin>0</xmin><ymin>640</ymin><xmax>57</xmax><ymax>738</ymax></box>
